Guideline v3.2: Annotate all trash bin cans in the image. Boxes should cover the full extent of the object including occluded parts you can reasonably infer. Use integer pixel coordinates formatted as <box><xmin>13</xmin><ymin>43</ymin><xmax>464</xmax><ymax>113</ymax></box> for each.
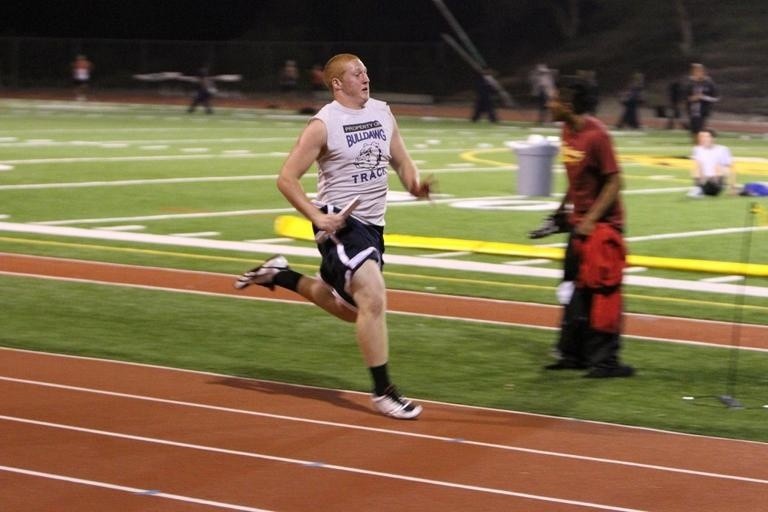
<box><xmin>515</xmin><ymin>144</ymin><xmax>558</xmax><ymax>197</ymax></box>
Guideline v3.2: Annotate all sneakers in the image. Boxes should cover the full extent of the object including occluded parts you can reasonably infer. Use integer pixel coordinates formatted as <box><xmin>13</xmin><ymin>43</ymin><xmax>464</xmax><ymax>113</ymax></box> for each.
<box><xmin>234</xmin><ymin>255</ymin><xmax>290</xmax><ymax>290</ymax></box>
<box><xmin>370</xmin><ymin>385</ymin><xmax>423</xmax><ymax>419</ymax></box>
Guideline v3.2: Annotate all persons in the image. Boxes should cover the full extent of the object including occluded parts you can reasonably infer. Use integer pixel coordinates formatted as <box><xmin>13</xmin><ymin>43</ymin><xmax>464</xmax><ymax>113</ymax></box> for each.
<box><xmin>685</xmin><ymin>127</ymin><xmax>736</xmax><ymax>198</ymax></box>
<box><xmin>234</xmin><ymin>52</ymin><xmax>441</xmax><ymax>419</ymax></box>
<box><xmin>71</xmin><ymin>53</ymin><xmax>99</xmax><ymax>102</ymax></box>
<box><xmin>566</xmin><ymin>65</ymin><xmax>599</xmax><ymax>114</ymax></box>
<box><xmin>186</xmin><ymin>64</ymin><xmax>218</xmax><ymax>115</ymax></box>
<box><xmin>527</xmin><ymin>62</ymin><xmax>559</xmax><ymax>123</ymax></box>
<box><xmin>614</xmin><ymin>72</ymin><xmax>646</xmax><ymax>129</ymax></box>
<box><xmin>683</xmin><ymin>62</ymin><xmax>722</xmax><ymax>144</ymax></box>
<box><xmin>470</xmin><ymin>66</ymin><xmax>500</xmax><ymax>123</ymax></box>
<box><xmin>541</xmin><ymin>75</ymin><xmax>636</xmax><ymax>378</ymax></box>
